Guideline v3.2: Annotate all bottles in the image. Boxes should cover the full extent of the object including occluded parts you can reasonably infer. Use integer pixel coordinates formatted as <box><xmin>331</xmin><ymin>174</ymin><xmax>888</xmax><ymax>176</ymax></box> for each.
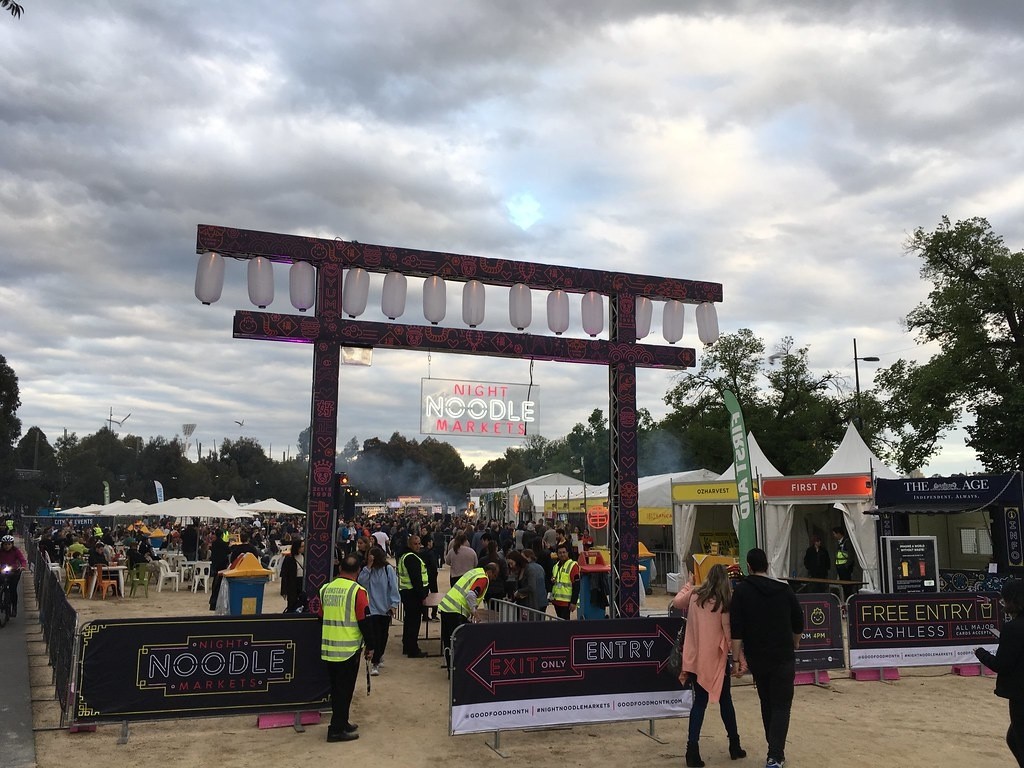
<box><xmin>295</xmin><ymin>606</ymin><xmax>305</xmax><ymax>614</ymax></box>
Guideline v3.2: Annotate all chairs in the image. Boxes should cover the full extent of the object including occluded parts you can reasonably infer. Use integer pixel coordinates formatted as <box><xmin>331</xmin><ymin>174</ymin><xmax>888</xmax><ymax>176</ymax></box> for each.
<box><xmin>42</xmin><ymin>545</ymin><xmax>212</xmax><ymax>595</ymax></box>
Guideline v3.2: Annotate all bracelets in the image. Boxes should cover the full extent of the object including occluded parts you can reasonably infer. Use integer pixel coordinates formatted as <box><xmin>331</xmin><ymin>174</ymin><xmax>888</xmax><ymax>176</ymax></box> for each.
<box><xmin>733</xmin><ymin>660</ymin><xmax>739</xmax><ymax>662</ymax></box>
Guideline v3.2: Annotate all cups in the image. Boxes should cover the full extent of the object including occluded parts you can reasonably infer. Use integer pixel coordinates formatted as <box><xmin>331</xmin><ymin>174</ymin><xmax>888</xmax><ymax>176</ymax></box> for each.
<box><xmin>114</xmin><ymin>562</ymin><xmax>118</xmax><ymax>566</ymax></box>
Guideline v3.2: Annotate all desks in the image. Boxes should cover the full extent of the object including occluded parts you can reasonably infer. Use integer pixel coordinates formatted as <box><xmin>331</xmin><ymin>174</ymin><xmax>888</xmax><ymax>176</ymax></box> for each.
<box><xmin>439</xmin><ymin>608</ymin><xmax>503</xmax><ymax>669</ymax></box>
<box><xmin>89</xmin><ymin>565</ymin><xmax>129</xmax><ymax>599</ymax></box>
<box><xmin>778</xmin><ymin>577</ymin><xmax>870</xmax><ymax>601</ymax></box>
<box><xmin>179</xmin><ymin>560</ymin><xmax>200</xmax><ymax>583</ymax></box>
<box><xmin>417</xmin><ymin>592</ymin><xmax>447</xmax><ymax>658</ymax></box>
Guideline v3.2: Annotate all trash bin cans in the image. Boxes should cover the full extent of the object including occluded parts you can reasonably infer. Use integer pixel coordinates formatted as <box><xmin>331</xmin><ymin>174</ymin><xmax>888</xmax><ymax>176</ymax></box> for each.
<box><xmin>638</xmin><ymin>542</ymin><xmax>656</xmax><ymax>594</ymax></box>
<box><xmin>140</xmin><ymin>525</ymin><xmax>150</xmax><ymax>539</ymax></box>
<box><xmin>127</xmin><ymin>524</ymin><xmax>134</xmax><ymax>531</ymax></box>
<box><xmin>218</xmin><ymin>551</ymin><xmax>275</xmax><ymax>615</ymax></box>
<box><xmin>577</xmin><ymin>550</ymin><xmax>611</xmax><ymax>620</ymax></box>
<box><xmin>148</xmin><ymin>527</ymin><xmax>165</xmax><ymax>548</ymax></box>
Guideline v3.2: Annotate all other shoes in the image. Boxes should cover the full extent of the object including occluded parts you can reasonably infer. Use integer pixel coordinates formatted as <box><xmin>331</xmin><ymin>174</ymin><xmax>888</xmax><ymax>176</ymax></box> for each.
<box><xmin>12</xmin><ymin>609</ymin><xmax>17</xmax><ymax>616</ymax></box>
<box><xmin>378</xmin><ymin>658</ymin><xmax>384</xmax><ymax>667</ymax></box>
<box><xmin>433</xmin><ymin>617</ymin><xmax>440</xmax><ymax>622</ymax></box>
<box><xmin>371</xmin><ymin>665</ymin><xmax>380</xmax><ymax>676</ymax></box>
<box><xmin>331</xmin><ymin>715</ymin><xmax>358</xmax><ymax>732</ymax></box>
<box><xmin>327</xmin><ymin>725</ymin><xmax>359</xmax><ymax>742</ymax></box>
<box><xmin>408</xmin><ymin>652</ymin><xmax>429</xmax><ymax>657</ymax></box>
<box><xmin>403</xmin><ymin>647</ymin><xmax>422</xmax><ymax>654</ymax></box>
<box><xmin>422</xmin><ymin>617</ymin><xmax>432</xmax><ymax>621</ymax></box>
<box><xmin>766</xmin><ymin>757</ymin><xmax>784</xmax><ymax>768</ymax></box>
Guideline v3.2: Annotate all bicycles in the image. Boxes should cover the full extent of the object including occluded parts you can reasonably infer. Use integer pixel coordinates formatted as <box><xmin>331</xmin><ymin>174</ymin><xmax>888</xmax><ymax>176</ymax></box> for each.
<box><xmin>0</xmin><ymin>564</ymin><xmax>24</xmax><ymax>628</ymax></box>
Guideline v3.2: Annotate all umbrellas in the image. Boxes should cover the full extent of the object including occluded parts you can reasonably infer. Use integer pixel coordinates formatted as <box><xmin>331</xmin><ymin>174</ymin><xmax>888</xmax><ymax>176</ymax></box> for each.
<box><xmin>56</xmin><ymin>494</ymin><xmax>306</xmax><ymax>560</ymax></box>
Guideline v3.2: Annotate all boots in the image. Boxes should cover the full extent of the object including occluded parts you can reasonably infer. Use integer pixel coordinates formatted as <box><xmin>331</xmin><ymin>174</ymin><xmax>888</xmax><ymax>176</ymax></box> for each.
<box><xmin>686</xmin><ymin>741</ymin><xmax>705</xmax><ymax>768</ymax></box>
<box><xmin>728</xmin><ymin>735</ymin><xmax>746</xmax><ymax>760</ymax></box>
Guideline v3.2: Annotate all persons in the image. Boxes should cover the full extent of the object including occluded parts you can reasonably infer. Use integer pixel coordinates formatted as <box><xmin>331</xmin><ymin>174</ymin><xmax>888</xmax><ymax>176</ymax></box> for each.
<box><xmin>398</xmin><ymin>536</ymin><xmax>429</xmax><ymax>658</ymax></box>
<box><xmin>673</xmin><ymin>563</ymin><xmax>747</xmax><ymax>767</ymax></box>
<box><xmin>319</xmin><ymin>557</ymin><xmax>376</xmax><ymax>743</ymax></box>
<box><xmin>729</xmin><ymin>548</ymin><xmax>804</xmax><ymax>768</ymax></box>
<box><xmin>804</xmin><ymin>537</ymin><xmax>831</xmax><ymax>594</ymax></box>
<box><xmin>550</xmin><ymin>548</ymin><xmax>581</xmax><ymax>619</ymax></box>
<box><xmin>974</xmin><ymin>581</ymin><xmax>1024</xmax><ymax>768</ymax></box>
<box><xmin>31</xmin><ymin>514</ymin><xmax>305</xmax><ymax>612</ymax></box>
<box><xmin>0</xmin><ymin>512</ymin><xmax>21</xmax><ymax>535</ymax></box>
<box><xmin>333</xmin><ymin>513</ymin><xmax>595</xmax><ymax>621</ymax></box>
<box><xmin>0</xmin><ymin>535</ymin><xmax>26</xmax><ymax>617</ymax></box>
<box><xmin>359</xmin><ymin>549</ymin><xmax>400</xmax><ymax>676</ymax></box>
<box><xmin>439</xmin><ymin>563</ymin><xmax>499</xmax><ymax>679</ymax></box>
<box><xmin>833</xmin><ymin>527</ymin><xmax>855</xmax><ymax>597</ymax></box>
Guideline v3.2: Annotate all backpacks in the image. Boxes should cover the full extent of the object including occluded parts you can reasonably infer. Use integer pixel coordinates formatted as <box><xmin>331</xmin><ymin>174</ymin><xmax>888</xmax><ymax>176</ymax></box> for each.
<box><xmin>342</xmin><ymin>528</ymin><xmax>349</xmax><ymax>540</ymax></box>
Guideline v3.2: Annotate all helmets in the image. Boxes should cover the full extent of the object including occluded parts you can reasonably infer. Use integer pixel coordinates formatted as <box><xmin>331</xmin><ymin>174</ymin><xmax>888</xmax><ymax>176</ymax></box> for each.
<box><xmin>2</xmin><ymin>535</ymin><xmax>14</xmax><ymax>543</ymax></box>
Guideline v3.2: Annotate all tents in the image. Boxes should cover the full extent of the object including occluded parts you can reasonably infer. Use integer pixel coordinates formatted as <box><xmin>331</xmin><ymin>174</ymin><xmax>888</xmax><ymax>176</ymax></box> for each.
<box><xmin>481</xmin><ymin>422</ymin><xmax>901</xmax><ymax>593</ymax></box>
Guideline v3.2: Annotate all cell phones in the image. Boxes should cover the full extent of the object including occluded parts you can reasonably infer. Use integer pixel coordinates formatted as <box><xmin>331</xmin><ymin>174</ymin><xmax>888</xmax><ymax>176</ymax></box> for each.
<box><xmin>989</xmin><ymin>629</ymin><xmax>1000</xmax><ymax>639</ymax></box>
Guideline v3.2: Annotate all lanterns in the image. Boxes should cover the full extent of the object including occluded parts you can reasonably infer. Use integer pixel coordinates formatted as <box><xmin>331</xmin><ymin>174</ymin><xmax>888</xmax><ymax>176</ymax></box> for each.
<box><xmin>636</xmin><ymin>295</ymin><xmax>719</xmax><ymax>346</ymax></box>
<box><xmin>196</xmin><ymin>249</ymin><xmax>316</xmax><ymax>312</ymax></box>
<box><xmin>343</xmin><ymin>266</ymin><xmax>605</xmax><ymax>337</ymax></box>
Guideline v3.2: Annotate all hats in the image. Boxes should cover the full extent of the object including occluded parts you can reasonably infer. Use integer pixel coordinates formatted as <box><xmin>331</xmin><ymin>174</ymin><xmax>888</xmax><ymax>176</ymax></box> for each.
<box><xmin>527</xmin><ymin>523</ymin><xmax>534</xmax><ymax>531</ymax></box>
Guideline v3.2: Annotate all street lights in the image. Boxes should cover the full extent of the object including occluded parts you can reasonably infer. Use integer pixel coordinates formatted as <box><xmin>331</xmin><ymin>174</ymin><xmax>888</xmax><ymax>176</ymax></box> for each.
<box><xmin>853</xmin><ymin>338</ymin><xmax>880</xmax><ymax>439</ymax></box>
